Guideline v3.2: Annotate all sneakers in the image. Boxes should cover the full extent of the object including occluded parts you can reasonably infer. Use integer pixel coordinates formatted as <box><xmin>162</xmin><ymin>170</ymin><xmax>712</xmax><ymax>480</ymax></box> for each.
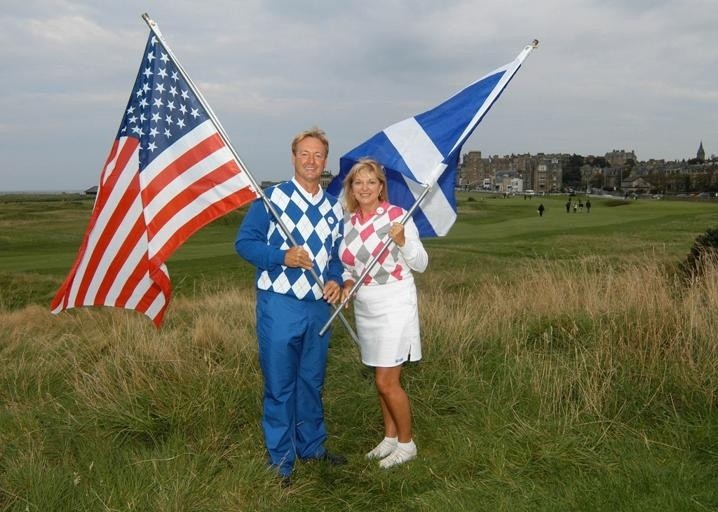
<box><xmin>364</xmin><ymin>436</ymin><xmax>417</xmax><ymax>468</ymax></box>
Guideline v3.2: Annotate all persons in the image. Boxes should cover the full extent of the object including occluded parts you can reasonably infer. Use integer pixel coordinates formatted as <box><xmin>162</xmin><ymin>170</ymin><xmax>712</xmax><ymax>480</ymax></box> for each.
<box><xmin>502</xmin><ymin>191</ymin><xmax>533</xmax><ymax>200</ymax></box>
<box><xmin>339</xmin><ymin>157</ymin><xmax>428</xmax><ymax>468</ymax></box>
<box><xmin>537</xmin><ymin>204</ymin><xmax>545</xmax><ymax>216</ymax></box>
<box><xmin>565</xmin><ymin>197</ymin><xmax>584</xmax><ymax>213</ymax></box>
<box><xmin>586</xmin><ymin>200</ymin><xmax>592</xmax><ymax>211</ymax></box>
<box><xmin>235</xmin><ymin>129</ymin><xmax>347</xmax><ymax>490</ymax></box>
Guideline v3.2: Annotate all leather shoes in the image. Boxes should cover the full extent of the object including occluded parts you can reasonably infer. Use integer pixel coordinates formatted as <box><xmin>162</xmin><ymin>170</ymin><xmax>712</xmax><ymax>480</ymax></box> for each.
<box><xmin>281</xmin><ymin>475</ymin><xmax>293</xmax><ymax>489</ymax></box>
<box><xmin>302</xmin><ymin>452</ymin><xmax>348</xmax><ymax>465</ymax></box>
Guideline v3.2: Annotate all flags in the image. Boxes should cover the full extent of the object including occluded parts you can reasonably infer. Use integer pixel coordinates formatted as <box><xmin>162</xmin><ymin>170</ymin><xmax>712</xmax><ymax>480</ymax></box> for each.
<box><xmin>326</xmin><ymin>40</ymin><xmax>540</xmax><ymax>236</ymax></box>
<box><xmin>50</xmin><ymin>13</ymin><xmax>265</xmax><ymax>334</ymax></box>
